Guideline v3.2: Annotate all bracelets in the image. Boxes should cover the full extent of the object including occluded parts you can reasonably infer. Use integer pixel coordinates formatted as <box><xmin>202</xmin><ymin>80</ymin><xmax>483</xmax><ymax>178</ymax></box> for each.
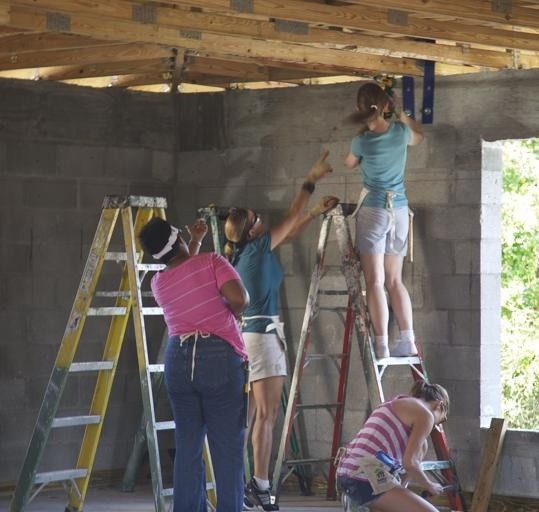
<box><xmin>190</xmin><ymin>238</ymin><xmax>201</xmax><ymax>247</ymax></box>
<box><xmin>301</xmin><ymin>178</ymin><xmax>315</xmax><ymax>194</ymax></box>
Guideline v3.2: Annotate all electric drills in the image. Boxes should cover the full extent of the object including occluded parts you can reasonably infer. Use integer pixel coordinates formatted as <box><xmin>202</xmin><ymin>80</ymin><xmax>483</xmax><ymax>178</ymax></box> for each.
<box><xmin>373</xmin><ymin>75</ymin><xmax>397</xmax><ymax>119</ymax></box>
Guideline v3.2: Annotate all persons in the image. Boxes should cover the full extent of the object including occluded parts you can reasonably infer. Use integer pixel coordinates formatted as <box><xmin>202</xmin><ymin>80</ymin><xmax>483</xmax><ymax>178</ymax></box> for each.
<box><xmin>344</xmin><ymin>82</ymin><xmax>426</xmax><ymax>359</ymax></box>
<box><xmin>334</xmin><ymin>381</ymin><xmax>453</xmax><ymax>512</ymax></box>
<box><xmin>218</xmin><ymin>148</ymin><xmax>336</xmax><ymax>511</ymax></box>
<box><xmin>137</xmin><ymin>210</ymin><xmax>251</xmax><ymax>512</ymax></box>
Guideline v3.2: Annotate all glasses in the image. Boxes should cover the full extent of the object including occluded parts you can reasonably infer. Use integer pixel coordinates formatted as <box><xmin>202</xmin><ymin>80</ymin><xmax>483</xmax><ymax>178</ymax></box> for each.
<box><xmin>246</xmin><ymin>213</ymin><xmax>260</xmax><ymax>231</ymax></box>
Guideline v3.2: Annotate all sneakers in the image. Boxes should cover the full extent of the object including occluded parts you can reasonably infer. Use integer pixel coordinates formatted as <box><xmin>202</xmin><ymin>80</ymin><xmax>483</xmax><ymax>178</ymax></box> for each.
<box><xmin>242</xmin><ymin>476</ymin><xmax>280</xmax><ymax>512</ymax></box>
<box><xmin>341</xmin><ymin>492</ymin><xmax>370</xmax><ymax>512</ymax></box>
<box><xmin>390</xmin><ymin>340</ymin><xmax>419</xmax><ymax>357</ymax></box>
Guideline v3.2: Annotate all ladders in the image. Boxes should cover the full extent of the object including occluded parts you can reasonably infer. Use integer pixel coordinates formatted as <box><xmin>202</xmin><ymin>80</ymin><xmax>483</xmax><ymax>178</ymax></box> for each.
<box><xmin>269</xmin><ymin>202</ymin><xmax>468</xmax><ymax>512</ymax></box>
<box><xmin>10</xmin><ymin>194</ymin><xmax>217</xmax><ymax>512</ymax></box>
<box><xmin>121</xmin><ymin>202</ymin><xmax>307</xmax><ymax>497</ymax></box>
